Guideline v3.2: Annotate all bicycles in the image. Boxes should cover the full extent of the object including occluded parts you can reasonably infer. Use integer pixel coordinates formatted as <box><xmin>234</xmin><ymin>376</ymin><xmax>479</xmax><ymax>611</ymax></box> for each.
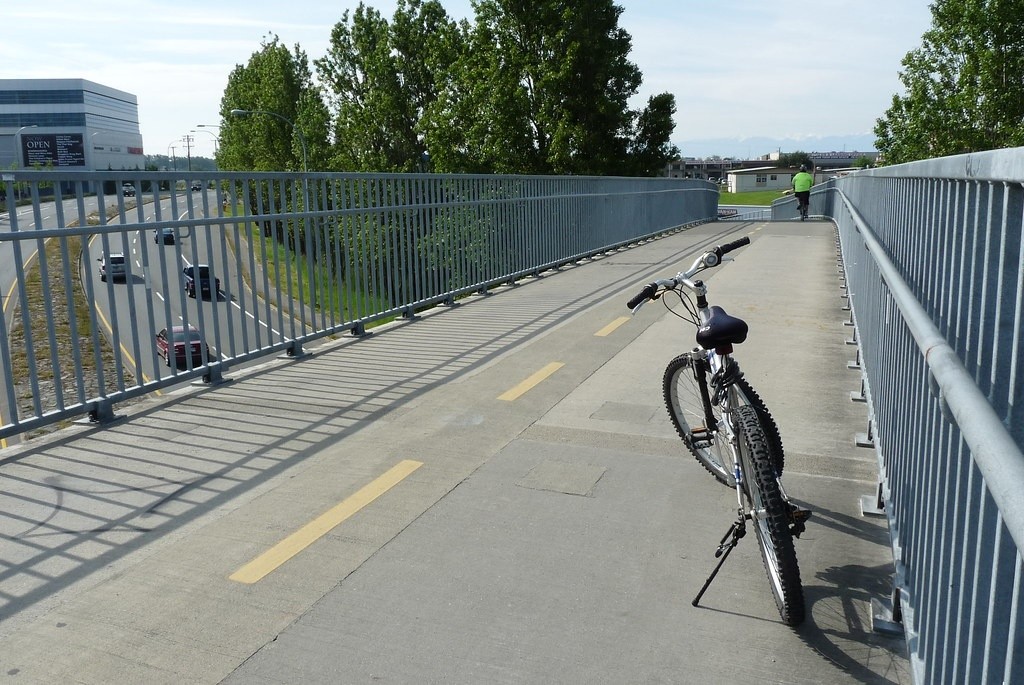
<box><xmin>625</xmin><ymin>236</ymin><xmax>814</xmax><ymax>628</ymax></box>
<box><xmin>793</xmin><ymin>185</ymin><xmax>812</xmax><ymax>222</ymax></box>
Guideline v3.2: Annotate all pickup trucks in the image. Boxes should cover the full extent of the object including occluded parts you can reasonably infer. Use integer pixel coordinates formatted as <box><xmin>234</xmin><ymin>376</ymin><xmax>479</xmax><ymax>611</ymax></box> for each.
<box><xmin>182</xmin><ymin>264</ymin><xmax>220</xmax><ymax>298</ymax></box>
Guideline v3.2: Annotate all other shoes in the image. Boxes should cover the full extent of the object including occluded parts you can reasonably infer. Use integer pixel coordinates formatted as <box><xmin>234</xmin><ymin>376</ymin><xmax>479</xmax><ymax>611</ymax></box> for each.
<box><xmin>805</xmin><ymin>209</ymin><xmax>808</xmax><ymax>218</ymax></box>
<box><xmin>797</xmin><ymin>202</ymin><xmax>801</xmax><ymax>209</ymax></box>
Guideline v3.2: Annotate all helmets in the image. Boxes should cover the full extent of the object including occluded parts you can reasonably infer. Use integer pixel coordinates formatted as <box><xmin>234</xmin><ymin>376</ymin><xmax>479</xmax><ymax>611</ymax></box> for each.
<box><xmin>799</xmin><ymin>165</ymin><xmax>807</xmax><ymax>172</ymax></box>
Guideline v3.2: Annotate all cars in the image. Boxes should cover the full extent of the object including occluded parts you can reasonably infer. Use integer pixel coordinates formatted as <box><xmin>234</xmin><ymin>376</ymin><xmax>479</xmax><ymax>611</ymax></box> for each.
<box><xmin>708</xmin><ymin>177</ymin><xmax>728</xmax><ymax>185</ymax></box>
<box><xmin>155</xmin><ymin>325</ymin><xmax>210</xmax><ymax>366</ymax></box>
<box><xmin>153</xmin><ymin>228</ymin><xmax>175</xmax><ymax>244</ymax></box>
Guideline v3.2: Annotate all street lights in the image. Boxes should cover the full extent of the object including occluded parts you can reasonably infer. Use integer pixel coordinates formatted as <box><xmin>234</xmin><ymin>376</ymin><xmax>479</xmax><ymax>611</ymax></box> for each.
<box><xmin>14</xmin><ymin>124</ymin><xmax>37</xmax><ymax>201</ymax></box>
<box><xmin>87</xmin><ymin>130</ymin><xmax>107</xmax><ymax>193</ymax></box>
<box><xmin>190</xmin><ymin>129</ymin><xmax>227</xmax><ymax>211</ymax></box>
<box><xmin>231</xmin><ymin>108</ymin><xmax>318</xmax><ymax>307</ymax></box>
<box><xmin>166</xmin><ymin>139</ymin><xmax>182</xmax><ymax>159</ymax></box>
<box><xmin>196</xmin><ymin>124</ymin><xmax>248</xmax><ymax>237</ymax></box>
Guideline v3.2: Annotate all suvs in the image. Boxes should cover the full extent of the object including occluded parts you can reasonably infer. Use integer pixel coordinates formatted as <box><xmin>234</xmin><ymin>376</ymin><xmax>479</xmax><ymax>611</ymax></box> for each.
<box><xmin>122</xmin><ymin>182</ymin><xmax>136</xmax><ymax>197</ymax></box>
<box><xmin>96</xmin><ymin>251</ymin><xmax>126</xmax><ymax>281</ymax></box>
<box><xmin>191</xmin><ymin>183</ymin><xmax>201</xmax><ymax>191</ymax></box>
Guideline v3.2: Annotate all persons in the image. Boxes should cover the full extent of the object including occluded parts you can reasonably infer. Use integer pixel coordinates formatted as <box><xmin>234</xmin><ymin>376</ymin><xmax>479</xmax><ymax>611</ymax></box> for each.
<box><xmin>791</xmin><ymin>164</ymin><xmax>813</xmax><ymax>210</ymax></box>
